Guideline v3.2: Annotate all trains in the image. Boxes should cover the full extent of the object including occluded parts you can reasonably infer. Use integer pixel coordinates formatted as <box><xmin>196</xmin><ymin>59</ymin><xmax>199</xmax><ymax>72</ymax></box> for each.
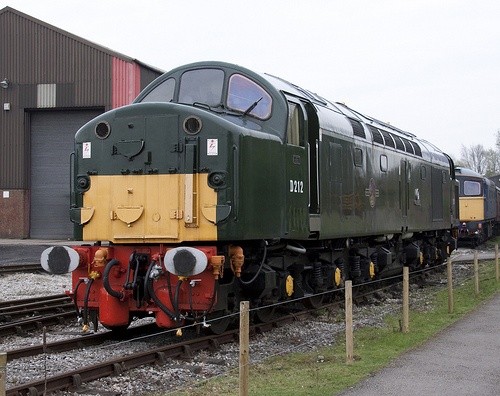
<box><xmin>39</xmin><ymin>59</ymin><xmax>500</xmax><ymax>334</ymax></box>
